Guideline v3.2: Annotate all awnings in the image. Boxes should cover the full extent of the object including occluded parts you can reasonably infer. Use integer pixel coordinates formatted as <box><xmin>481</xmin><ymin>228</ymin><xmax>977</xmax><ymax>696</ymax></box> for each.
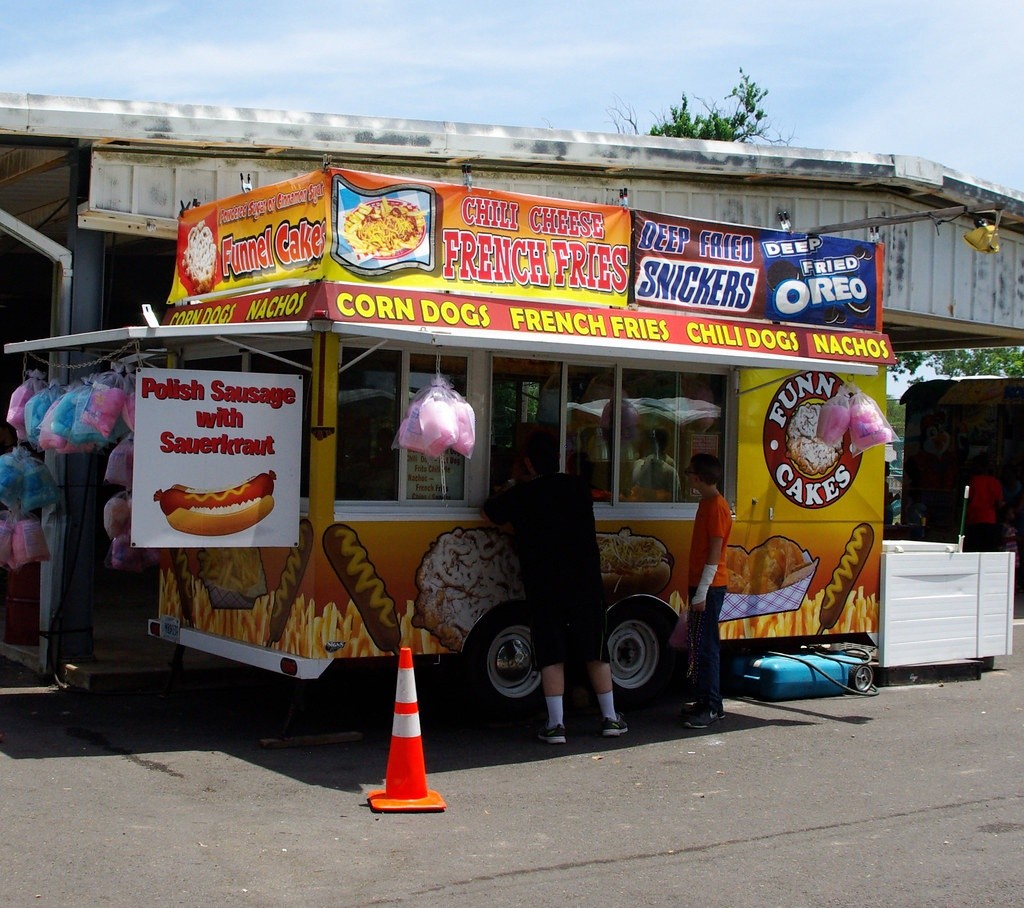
<box><xmin>334</xmin><ymin>319</ymin><xmax>878</xmax><ymax>380</ymax></box>
<box><xmin>5</xmin><ymin>317</ymin><xmax>313</xmax><ymax>371</ymax></box>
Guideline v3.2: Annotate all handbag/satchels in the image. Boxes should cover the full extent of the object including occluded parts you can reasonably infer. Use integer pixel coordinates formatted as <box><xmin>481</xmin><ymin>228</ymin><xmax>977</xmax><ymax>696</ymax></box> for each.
<box><xmin>669</xmin><ymin>607</ymin><xmax>692</xmax><ymax>649</ymax></box>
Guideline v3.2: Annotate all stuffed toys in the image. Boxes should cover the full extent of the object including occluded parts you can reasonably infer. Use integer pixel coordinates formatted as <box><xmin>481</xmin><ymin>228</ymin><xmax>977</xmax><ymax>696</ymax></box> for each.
<box><xmin>0</xmin><ymin>368</ymin><xmax>169</xmax><ymax>570</ymax></box>
<box><xmin>397</xmin><ymin>388</ymin><xmax>476</xmax><ymax>456</ymax></box>
<box><xmin>815</xmin><ymin>394</ymin><xmax>893</xmax><ymax>454</ymax></box>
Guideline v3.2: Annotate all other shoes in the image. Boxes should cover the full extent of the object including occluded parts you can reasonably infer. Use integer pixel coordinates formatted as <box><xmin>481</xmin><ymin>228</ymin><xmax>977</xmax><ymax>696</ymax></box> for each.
<box><xmin>1016</xmin><ymin>584</ymin><xmax>1024</xmax><ymax>592</ymax></box>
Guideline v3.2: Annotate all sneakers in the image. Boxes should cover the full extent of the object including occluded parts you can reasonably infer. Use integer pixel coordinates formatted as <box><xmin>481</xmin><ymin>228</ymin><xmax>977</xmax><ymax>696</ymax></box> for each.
<box><xmin>685</xmin><ymin>708</ymin><xmax>725</xmax><ymax>727</ymax></box>
<box><xmin>601</xmin><ymin>711</ymin><xmax>628</xmax><ymax>736</ymax></box>
<box><xmin>538</xmin><ymin>718</ymin><xmax>567</xmax><ymax>743</ymax></box>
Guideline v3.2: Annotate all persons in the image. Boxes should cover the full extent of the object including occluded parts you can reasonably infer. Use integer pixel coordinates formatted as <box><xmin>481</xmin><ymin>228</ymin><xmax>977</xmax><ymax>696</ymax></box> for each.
<box><xmin>480</xmin><ymin>431</ymin><xmax>628</xmax><ymax>744</ymax></box>
<box><xmin>966</xmin><ymin>461</ymin><xmax>1024</xmax><ymax>594</ymax></box>
<box><xmin>884</xmin><ymin>462</ymin><xmax>901</xmax><ymax>525</ymax></box>
<box><xmin>680</xmin><ymin>453</ymin><xmax>732</xmax><ymax>728</ymax></box>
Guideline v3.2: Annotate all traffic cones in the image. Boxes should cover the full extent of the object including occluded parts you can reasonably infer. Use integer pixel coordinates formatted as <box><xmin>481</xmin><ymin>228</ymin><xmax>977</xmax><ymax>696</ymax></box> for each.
<box><xmin>365</xmin><ymin>644</ymin><xmax>445</xmax><ymax>812</ymax></box>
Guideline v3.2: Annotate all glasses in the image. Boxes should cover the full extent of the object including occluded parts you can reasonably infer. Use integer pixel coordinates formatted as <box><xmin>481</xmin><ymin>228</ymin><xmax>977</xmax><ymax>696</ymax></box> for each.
<box><xmin>684</xmin><ymin>467</ymin><xmax>696</xmax><ymax>475</ymax></box>
